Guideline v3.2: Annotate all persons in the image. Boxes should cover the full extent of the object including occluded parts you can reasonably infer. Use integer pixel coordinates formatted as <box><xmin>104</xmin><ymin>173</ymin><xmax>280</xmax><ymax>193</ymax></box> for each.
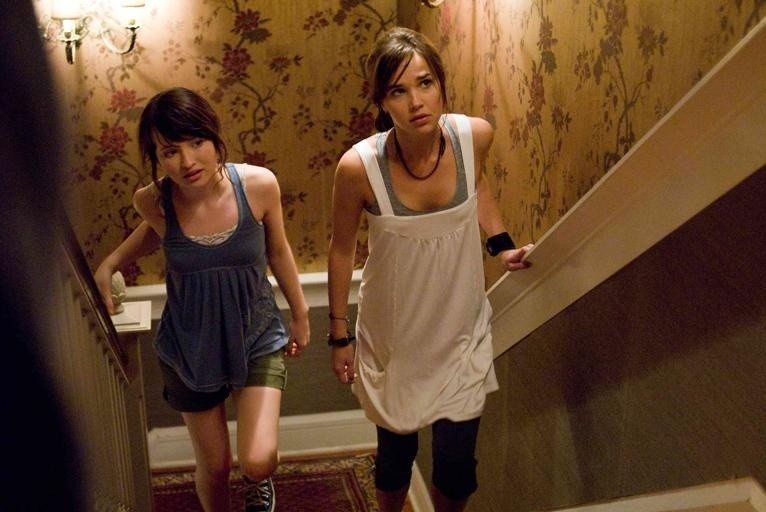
<box><xmin>328</xmin><ymin>29</ymin><xmax>535</xmax><ymax>511</ymax></box>
<box><xmin>92</xmin><ymin>87</ymin><xmax>312</xmax><ymax>511</ymax></box>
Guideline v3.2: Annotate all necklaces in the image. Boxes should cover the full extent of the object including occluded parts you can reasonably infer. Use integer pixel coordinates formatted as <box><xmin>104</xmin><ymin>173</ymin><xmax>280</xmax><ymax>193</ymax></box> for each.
<box><xmin>394</xmin><ymin>127</ymin><xmax>447</xmax><ymax>179</ymax></box>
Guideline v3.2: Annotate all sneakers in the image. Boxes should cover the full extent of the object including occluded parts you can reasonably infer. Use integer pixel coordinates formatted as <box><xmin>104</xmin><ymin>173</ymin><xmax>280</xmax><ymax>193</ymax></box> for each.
<box><xmin>243</xmin><ymin>473</ymin><xmax>276</xmax><ymax>511</ymax></box>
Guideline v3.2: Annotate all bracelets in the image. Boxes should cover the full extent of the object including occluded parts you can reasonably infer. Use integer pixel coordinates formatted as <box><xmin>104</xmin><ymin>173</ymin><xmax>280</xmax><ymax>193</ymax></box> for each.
<box><xmin>328</xmin><ymin>332</ymin><xmax>356</xmax><ymax>348</ymax></box>
<box><xmin>329</xmin><ymin>312</ymin><xmax>351</xmax><ymax>323</ymax></box>
<box><xmin>486</xmin><ymin>232</ymin><xmax>518</xmax><ymax>256</ymax></box>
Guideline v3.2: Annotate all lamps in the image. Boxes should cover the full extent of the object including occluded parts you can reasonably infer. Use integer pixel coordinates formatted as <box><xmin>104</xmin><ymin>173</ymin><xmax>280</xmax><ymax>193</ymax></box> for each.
<box><xmin>31</xmin><ymin>0</ymin><xmax>149</xmax><ymax>66</ymax></box>
